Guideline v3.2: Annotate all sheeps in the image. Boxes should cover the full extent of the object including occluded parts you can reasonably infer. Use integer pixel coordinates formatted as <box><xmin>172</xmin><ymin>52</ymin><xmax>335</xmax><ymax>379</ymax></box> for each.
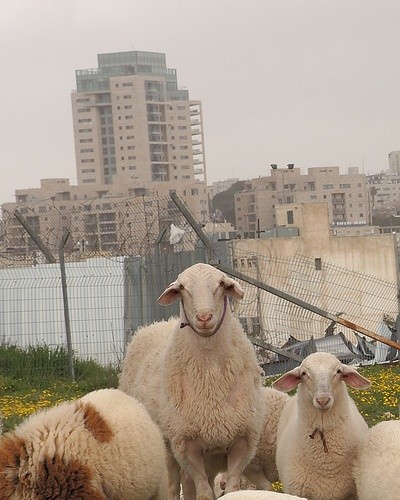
<box><xmin>1</xmin><ymin>388</ymin><xmax>169</xmax><ymax>499</ymax></box>
<box><xmin>117</xmin><ymin>252</ymin><xmax>290</xmax><ymax>500</ymax></box>
<box><xmin>272</xmin><ymin>352</ymin><xmax>400</xmax><ymax>500</ymax></box>
